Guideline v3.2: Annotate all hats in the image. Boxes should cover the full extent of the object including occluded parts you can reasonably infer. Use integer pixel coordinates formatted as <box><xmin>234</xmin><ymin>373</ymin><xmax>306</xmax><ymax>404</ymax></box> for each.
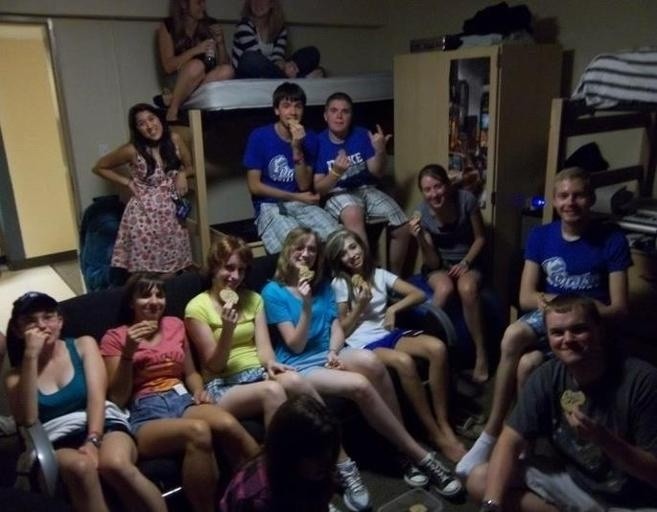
<box><xmin>14</xmin><ymin>293</ymin><xmax>58</xmax><ymax>314</ymax></box>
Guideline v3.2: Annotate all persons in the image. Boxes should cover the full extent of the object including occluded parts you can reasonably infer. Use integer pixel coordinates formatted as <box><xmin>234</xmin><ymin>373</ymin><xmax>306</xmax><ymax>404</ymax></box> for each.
<box><xmin>404</xmin><ymin>162</ymin><xmax>492</xmax><ymax>384</ymax></box>
<box><xmin>463</xmin><ymin>293</ymin><xmax>656</xmax><ymax>512</ymax></box>
<box><xmin>152</xmin><ymin>1</ymin><xmax>240</xmax><ymax>122</ymax></box>
<box><xmin>241</xmin><ymin>81</ymin><xmax>347</xmax><ymax>256</ymax></box>
<box><xmin>454</xmin><ymin>166</ymin><xmax>636</xmax><ymax>477</ymax></box>
<box><xmin>231</xmin><ymin>0</ymin><xmax>327</xmax><ymax>81</ymax></box>
<box><xmin>91</xmin><ymin>102</ymin><xmax>195</xmax><ymax>288</ymax></box>
<box><xmin>312</xmin><ymin>91</ymin><xmax>411</xmax><ymax>282</ymax></box>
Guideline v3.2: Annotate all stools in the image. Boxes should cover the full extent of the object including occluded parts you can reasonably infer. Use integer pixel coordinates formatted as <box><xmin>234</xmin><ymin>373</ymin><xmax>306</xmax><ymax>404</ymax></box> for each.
<box><xmin>402</xmin><ymin>272</ymin><xmax>501</xmax><ymax>377</ymax></box>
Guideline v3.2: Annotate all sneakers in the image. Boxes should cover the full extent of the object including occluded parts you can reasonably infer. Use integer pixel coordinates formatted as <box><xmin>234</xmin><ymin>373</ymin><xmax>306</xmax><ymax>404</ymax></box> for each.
<box><xmin>398</xmin><ymin>454</ymin><xmax>430</xmax><ymax>486</ymax></box>
<box><xmin>418</xmin><ymin>452</ymin><xmax>462</xmax><ymax>496</ymax></box>
<box><xmin>333</xmin><ymin>459</ymin><xmax>372</xmax><ymax>512</ymax></box>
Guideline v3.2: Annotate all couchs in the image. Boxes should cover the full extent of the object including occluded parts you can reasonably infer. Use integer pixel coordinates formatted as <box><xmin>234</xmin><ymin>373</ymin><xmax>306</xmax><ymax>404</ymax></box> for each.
<box><xmin>5</xmin><ymin>240</ymin><xmax>459</xmax><ymax>512</ymax></box>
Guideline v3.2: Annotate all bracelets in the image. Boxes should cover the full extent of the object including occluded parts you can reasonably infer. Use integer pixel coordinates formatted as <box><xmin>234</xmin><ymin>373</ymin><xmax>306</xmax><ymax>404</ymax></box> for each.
<box><xmin>480</xmin><ymin>500</ymin><xmax>500</xmax><ymax>512</ymax></box>
<box><xmin>331</xmin><ymin>170</ymin><xmax>342</xmax><ymax>177</ymax></box>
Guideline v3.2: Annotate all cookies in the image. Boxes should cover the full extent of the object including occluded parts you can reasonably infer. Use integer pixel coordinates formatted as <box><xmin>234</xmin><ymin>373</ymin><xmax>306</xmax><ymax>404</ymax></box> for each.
<box><xmin>219</xmin><ymin>287</ymin><xmax>239</xmax><ymax>305</ymax></box>
<box><xmin>557</xmin><ymin>390</ymin><xmax>585</xmax><ymax>414</ymax></box>
<box><xmin>300</xmin><ymin>265</ymin><xmax>316</xmax><ymax>280</ymax></box>
<box><xmin>147</xmin><ymin>320</ymin><xmax>159</xmax><ymax>334</ymax></box>
<box><xmin>407</xmin><ymin>502</ymin><xmax>430</xmax><ymax>512</ymax></box>
<box><xmin>287</xmin><ymin>117</ymin><xmax>299</xmax><ymax>126</ymax></box>
<box><xmin>349</xmin><ymin>273</ymin><xmax>363</xmax><ymax>287</ymax></box>
<box><xmin>414</xmin><ymin>210</ymin><xmax>422</xmax><ymax>217</ymax></box>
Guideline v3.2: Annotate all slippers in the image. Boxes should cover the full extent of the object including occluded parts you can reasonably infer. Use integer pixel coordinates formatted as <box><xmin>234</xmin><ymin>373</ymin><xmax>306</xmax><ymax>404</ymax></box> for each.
<box><xmin>454</xmin><ymin>415</ymin><xmax>487</xmax><ymax>440</ymax></box>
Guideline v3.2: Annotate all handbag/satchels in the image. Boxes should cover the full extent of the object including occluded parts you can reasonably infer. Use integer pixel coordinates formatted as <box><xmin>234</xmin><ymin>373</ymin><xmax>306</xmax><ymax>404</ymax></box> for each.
<box><xmin>464</xmin><ymin>2</ymin><xmax>533</xmax><ymax>36</ymax></box>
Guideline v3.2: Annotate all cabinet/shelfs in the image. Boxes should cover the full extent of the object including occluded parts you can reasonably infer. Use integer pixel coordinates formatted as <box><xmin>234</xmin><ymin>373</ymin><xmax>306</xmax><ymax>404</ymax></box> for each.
<box><xmin>392</xmin><ymin>42</ymin><xmax>565</xmax><ymax>323</ymax></box>
<box><xmin>540</xmin><ymin>95</ymin><xmax>655</xmax><ymax>364</ymax></box>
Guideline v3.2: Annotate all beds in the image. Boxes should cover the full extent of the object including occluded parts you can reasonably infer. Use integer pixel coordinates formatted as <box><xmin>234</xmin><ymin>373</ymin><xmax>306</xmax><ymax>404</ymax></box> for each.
<box><xmin>159</xmin><ymin>69</ymin><xmax>394</xmax><ymax>289</ymax></box>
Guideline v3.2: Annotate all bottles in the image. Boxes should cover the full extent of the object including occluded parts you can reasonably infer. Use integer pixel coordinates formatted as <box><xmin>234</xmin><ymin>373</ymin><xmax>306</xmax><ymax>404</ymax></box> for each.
<box><xmin>204</xmin><ymin>36</ymin><xmax>216</xmax><ymax>70</ymax></box>
